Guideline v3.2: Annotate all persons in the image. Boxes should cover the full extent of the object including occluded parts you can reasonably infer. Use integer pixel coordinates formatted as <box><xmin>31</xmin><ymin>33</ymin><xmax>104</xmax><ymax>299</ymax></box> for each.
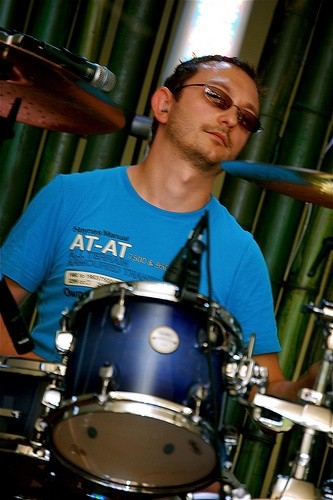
<box><xmin>0</xmin><ymin>54</ymin><xmax>333</xmax><ymax>403</ymax></box>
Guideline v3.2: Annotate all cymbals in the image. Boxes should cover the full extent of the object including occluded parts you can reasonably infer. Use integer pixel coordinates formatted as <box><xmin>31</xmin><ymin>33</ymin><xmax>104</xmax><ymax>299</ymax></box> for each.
<box><xmin>219</xmin><ymin>156</ymin><xmax>333</xmax><ymax>210</ymax></box>
<box><xmin>0</xmin><ymin>30</ymin><xmax>127</xmax><ymax>138</ymax></box>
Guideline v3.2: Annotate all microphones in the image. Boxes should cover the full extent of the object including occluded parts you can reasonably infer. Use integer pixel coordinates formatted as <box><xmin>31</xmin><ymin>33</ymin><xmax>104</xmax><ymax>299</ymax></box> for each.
<box><xmin>164</xmin><ymin>214</ymin><xmax>208</xmax><ymax>286</ymax></box>
<box><xmin>11</xmin><ymin>33</ymin><xmax>117</xmax><ymax>94</ymax></box>
<box><xmin>0</xmin><ymin>279</ymin><xmax>35</xmax><ymax>355</ymax></box>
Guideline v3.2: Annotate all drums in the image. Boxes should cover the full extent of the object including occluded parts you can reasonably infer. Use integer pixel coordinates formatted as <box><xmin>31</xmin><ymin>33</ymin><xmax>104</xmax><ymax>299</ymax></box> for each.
<box><xmin>1</xmin><ymin>356</ymin><xmax>95</xmax><ymax>500</ymax></box>
<box><xmin>42</xmin><ymin>281</ymin><xmax>245</xmax><ymax>496</ymax></box>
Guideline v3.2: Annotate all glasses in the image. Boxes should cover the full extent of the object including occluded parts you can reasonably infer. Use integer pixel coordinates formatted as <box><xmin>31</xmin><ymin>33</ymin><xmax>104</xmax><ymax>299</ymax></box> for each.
<box><xmin>181</xmin><ymin>84</ymin><xmax>264</xmax><ymax>134</ymax></box>
